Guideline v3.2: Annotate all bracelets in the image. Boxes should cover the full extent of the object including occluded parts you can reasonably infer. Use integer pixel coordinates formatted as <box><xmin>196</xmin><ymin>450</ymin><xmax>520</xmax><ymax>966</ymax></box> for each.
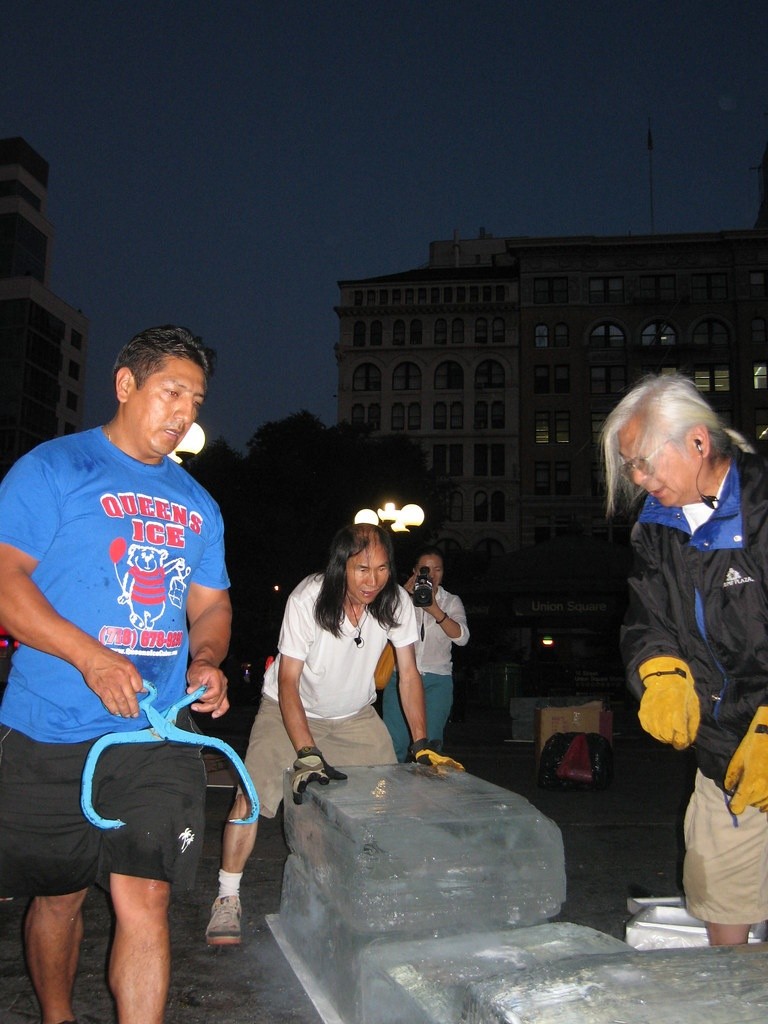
<box><xmin>436</xmin><ymin>613</ymin><xmax>448</xmax><ymax>623</ymax></box>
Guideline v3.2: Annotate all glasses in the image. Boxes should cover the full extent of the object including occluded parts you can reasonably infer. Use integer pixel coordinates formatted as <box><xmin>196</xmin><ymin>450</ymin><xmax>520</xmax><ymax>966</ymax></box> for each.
<box><xmin>619</xmin><ymin>438</ymin><xmax>676</xmax><ymax>483</ymax></box>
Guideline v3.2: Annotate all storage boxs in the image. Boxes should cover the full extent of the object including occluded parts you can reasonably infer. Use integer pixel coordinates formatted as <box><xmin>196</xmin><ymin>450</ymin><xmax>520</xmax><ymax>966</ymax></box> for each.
<box><xmin>535</xmin><ymin>701</ymin><xmax>613</xmax><ymax>752</ymax></box>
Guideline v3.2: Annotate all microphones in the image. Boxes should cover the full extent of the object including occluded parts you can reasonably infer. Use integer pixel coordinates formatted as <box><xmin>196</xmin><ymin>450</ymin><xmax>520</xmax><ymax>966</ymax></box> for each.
<box><xmin>354</xmin><ymin>638</ymin><xmax>361</xmax><ymax>644</ymax></box>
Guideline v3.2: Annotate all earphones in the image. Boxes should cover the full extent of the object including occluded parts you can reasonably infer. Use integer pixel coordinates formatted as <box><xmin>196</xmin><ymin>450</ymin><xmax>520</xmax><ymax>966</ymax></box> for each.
<box><xmin>695</xmin><ymin>439</ymin><xmax>704</xmax><ymax>454</ymax></box>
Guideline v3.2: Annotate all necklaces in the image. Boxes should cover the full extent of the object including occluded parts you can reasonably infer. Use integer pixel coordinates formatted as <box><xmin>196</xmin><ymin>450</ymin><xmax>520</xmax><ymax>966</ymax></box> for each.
<box><xmin>347</xmin><ymin>594</ymin><xmax>372</xmax><ymax>648</ymax></box>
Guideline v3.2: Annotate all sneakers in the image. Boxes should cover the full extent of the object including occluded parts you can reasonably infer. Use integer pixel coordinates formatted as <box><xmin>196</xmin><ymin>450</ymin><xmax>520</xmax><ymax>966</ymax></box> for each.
<box><xmin>205</xmin><ymin>894</ymin><xmax>243</xmax><ymax>944</ymax></box>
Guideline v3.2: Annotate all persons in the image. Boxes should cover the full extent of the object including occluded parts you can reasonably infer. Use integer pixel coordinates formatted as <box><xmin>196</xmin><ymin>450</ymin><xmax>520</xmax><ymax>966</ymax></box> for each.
<box><xmin>381</xmin><ymin>547</ymin><xmax>469</xmax><ymax>762</ymax></box>
<box><xmin>600</xmin><ymin>371</ymin><xmax>768</xmax><ymax>944</ymax></box>
<box><xmin>1</xmin><ymin>325</ymin><xmax>233</xmax><ymax>1024</ymax></box>
<box><xmin>203</xmin><ymin>523</ymin><xmax>463</xmax><ymax>944</ymax></box>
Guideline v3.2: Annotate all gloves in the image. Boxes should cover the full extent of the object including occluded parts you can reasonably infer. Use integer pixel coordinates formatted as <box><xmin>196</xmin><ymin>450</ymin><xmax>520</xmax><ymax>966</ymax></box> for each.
<box><xmin>637</xmin><ymin>654</ymin><xmax>698</xmax><ymax>748</ymax></box>
<box><xmin>290</xmin><ymin>748</ymin><xmax>347</xmax><ymax>804</ymax></box>
<box><xmin>724</xmin><ymin>706</ymin><xmax>768</xmax><ymax>814</ymax></box>
<box><xmin>410</xmin><ymin>738</ymin><xmax>463</xmax><ymax>774</ymax></box>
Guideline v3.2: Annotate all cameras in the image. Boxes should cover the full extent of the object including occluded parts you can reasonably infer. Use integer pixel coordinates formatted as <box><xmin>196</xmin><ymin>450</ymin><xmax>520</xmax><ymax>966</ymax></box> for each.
<box><xmin>412</xmin><ymin>566</ymin><xmax>433</xmax><ymax>607</ymax></box>
